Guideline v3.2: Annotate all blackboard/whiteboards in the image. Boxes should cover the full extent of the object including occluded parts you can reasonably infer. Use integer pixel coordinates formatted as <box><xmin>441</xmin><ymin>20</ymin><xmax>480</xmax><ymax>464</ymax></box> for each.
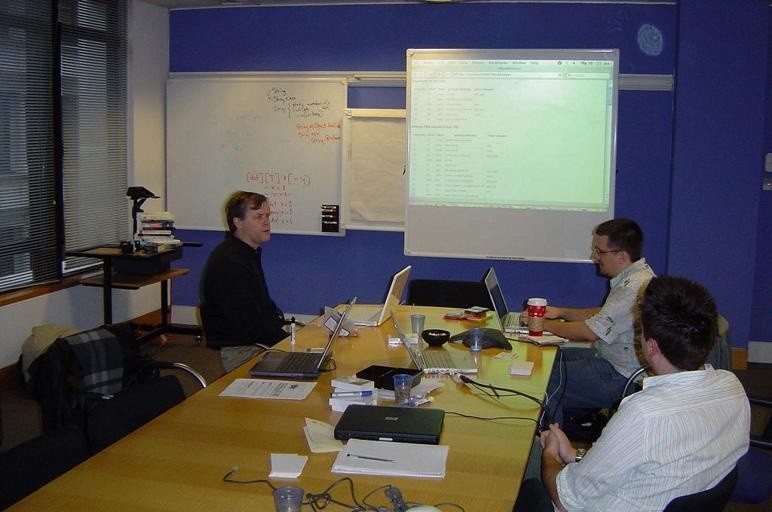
<box><xmin>164</xmin><ymin>77</ymin><xmax>348</xmax><ymax>237</ymax></box>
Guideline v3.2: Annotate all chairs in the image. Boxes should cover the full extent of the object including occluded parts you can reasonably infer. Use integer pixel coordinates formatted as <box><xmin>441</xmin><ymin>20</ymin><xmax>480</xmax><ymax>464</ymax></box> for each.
<box><xmin>731</xmin><ymin>394</ymin><xmax>772</xmax><ymax>504</ymax></box>
<box><xmin>31</xmin><ymin>321</ymin><xmax>209</xmax><ymax>478</ymax></box>
<box><xmin>406</xmin><ymin>279</ymin><xmax>494</xmax><ymax>312</ymax></box>
<box><xmin>562</xmin><ymin>365</ymin><xmax>654</xmax><ymax>445</ymax></box>
<box><xmin>663</xmin><ymin>464</ymin><xmax>737</xmax><ymax>512</ymax></box>
<box><xmin>196</xmin><ymin>303</ymin><xmax>306</xmax><ymax>377</ymax></box>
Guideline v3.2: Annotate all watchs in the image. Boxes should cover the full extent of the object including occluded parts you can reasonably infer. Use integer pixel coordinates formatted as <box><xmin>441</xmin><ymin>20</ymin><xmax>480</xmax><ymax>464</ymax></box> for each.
<box><xmin>575</xmin><ymin>447</ymin><xmax>586</xmax><ymax>462</ymax></box>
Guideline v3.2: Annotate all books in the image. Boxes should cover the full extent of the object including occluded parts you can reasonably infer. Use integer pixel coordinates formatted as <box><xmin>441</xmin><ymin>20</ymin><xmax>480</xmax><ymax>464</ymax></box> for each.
<box><xmin>138</xmin><ymin>212</ymin><xmax>176</xmax><ymax>244</ymax></box>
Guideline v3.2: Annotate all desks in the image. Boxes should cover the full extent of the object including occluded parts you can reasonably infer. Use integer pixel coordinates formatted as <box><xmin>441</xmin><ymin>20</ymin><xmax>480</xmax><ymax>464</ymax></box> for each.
<box><xmin>65</xmin><ymin>241</ymin><xmax>203</xmax><ymax>346</ymax></box>
<box><xmin>1</xmin><ymin>304</ymin><xmax>563</xmax><ymax>512</ymax></box>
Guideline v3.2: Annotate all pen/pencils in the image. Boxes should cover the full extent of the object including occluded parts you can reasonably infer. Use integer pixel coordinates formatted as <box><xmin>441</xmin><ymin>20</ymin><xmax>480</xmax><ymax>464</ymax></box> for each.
<box><xmin>291</xmin><ymin>317</ymin><xmax>295</xmax><ymax>345</ymax></box>
<box><xmin>331</xmin><ymin>391</ymin><xmax>372</xmax><ymax>399</ymax></box>
<box><xmin>347</xmin><ymin>453</ymin><xmax>396</xmax><ymax>462</ymax></box>
<box><xmin>403</xmin><ymin>396</ymin><xmax>434</xmax><ymax>407</ymax></box>
<box><xmin>489</xmin><ymin>383</ymin><xmax>499</xmax><ymax>397</ymax></box>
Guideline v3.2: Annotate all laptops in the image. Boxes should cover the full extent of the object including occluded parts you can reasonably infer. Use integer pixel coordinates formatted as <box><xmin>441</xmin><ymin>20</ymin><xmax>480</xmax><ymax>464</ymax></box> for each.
<box><xmin>247</xmin><ymin>295</ymin><xmax>358</xmax><ymax>380</ymax></box>
<box><xmin>354</xmin><ymin>264</ymin><xmax>413</xmax><ymax>328</ymax></box>
<box><xmin>483</xmin><ymin>265</ymin><xmax>553</xmax><ymax>336</ymax></box>
<box><xmin>389</xmin><ymin>310</ymin><xmax>477</xmax><ymax>375</ymax></box>
<box><xmin>334</xmin><ymin>404</ymin><xmax>445</xmax><ymax>445</ymax></box>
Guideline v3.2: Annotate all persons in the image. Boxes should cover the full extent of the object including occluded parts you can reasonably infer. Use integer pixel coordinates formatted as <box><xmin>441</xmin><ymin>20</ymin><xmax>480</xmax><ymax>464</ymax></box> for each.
<box><xmin>199</xmin><ymin>191</ymin><xmax>291</xmax><ymax>374</ymax></box>
<box><xmin>520</xmin><ymin>218</ymin><xmax>663</xmax><ymax>431</ymax></box>
<box><xmin>516</xmin><ymin>276</ymin><xmax>751</xmax><ymax>512</ymax></box>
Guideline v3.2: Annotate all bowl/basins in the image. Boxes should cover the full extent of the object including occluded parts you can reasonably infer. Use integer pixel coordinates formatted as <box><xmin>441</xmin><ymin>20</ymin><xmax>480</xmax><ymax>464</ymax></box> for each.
<box><xmin>422</xmin><ymin>329</ymin><xmax>450</xmax><ymax>348</ymax></box>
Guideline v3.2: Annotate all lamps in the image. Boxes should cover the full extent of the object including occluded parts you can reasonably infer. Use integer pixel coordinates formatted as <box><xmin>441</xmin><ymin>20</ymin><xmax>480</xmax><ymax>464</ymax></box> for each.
<box><xmin>127</xmin><ymin>184</ymin><xmax>159</xmax><ymax>242</ymax></box>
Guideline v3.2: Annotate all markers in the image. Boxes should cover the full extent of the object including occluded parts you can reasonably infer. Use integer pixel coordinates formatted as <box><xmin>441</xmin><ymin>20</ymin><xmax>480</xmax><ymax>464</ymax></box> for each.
<box><xmin>321</xmin><ymin>206</ymin><xmax>338</xmax><ymax>225</ymax></box>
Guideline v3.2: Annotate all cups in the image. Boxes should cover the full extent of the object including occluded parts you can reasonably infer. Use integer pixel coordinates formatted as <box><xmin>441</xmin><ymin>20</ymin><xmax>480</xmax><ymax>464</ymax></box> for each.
<box><xmin>272</xmin><ymin>485</ymin><xmax>304</xmax><ymax>512</ymax></box>
<box><xmin>527</xmin><ymin>297</ymin><xmax>547</xmax><ymax>336</ymax></box>
<box><xmin>469</xmin><ymin>328</ymin><xmax>484</xmax><ymax>352</ymax></box>
<box><xmin>394</xmin><ymin>375</ymin><xmax>413</xmax><ymax>403</ymax></box>
<box><xmin>410</xmin><ymin>314</ymin><xmax>425</xmax><ymax>336</ymax></box>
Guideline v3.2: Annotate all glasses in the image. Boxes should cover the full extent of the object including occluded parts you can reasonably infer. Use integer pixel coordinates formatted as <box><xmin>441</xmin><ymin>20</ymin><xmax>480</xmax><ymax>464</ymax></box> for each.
<box><xmin>593</xmin><ymin>245</ymin><xmax>632</xmax><ymax>258</ymax></box>
